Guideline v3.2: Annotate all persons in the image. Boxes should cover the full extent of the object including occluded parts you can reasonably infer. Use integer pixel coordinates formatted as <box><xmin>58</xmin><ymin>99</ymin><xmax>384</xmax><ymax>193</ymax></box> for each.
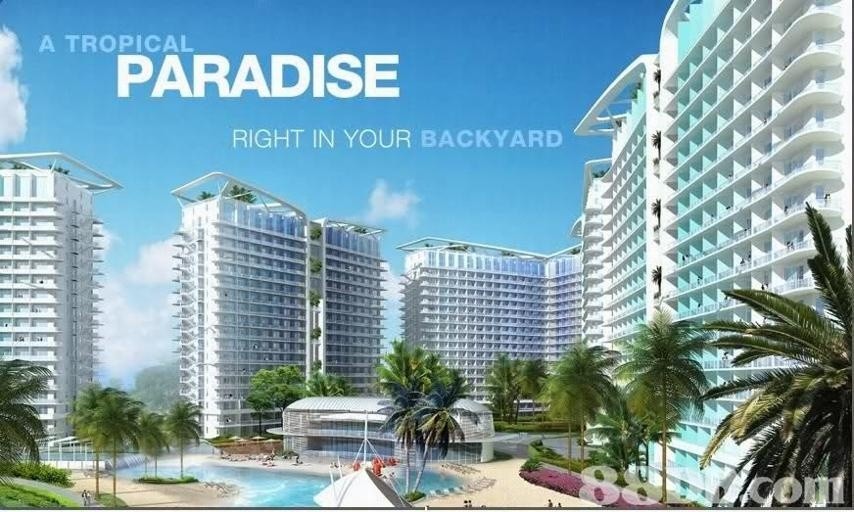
<box><xmin>85</xmin><ymin>491</ymin><xmax>92</xmax><ymax>505</ymax></box>
<box><xmin>82</xmin><ymin>489</ymin><xmax>87</xmax><ymax>507</ymax></box>
<box><xmin>465</xmin><ymin>497</ymin><xmax>562</xmax><ymax>508</ymax></box>
<box><xmin>211</xmin><ymin>447</ymin><xmax>397</xmax><ymax>478</ymax></box>
